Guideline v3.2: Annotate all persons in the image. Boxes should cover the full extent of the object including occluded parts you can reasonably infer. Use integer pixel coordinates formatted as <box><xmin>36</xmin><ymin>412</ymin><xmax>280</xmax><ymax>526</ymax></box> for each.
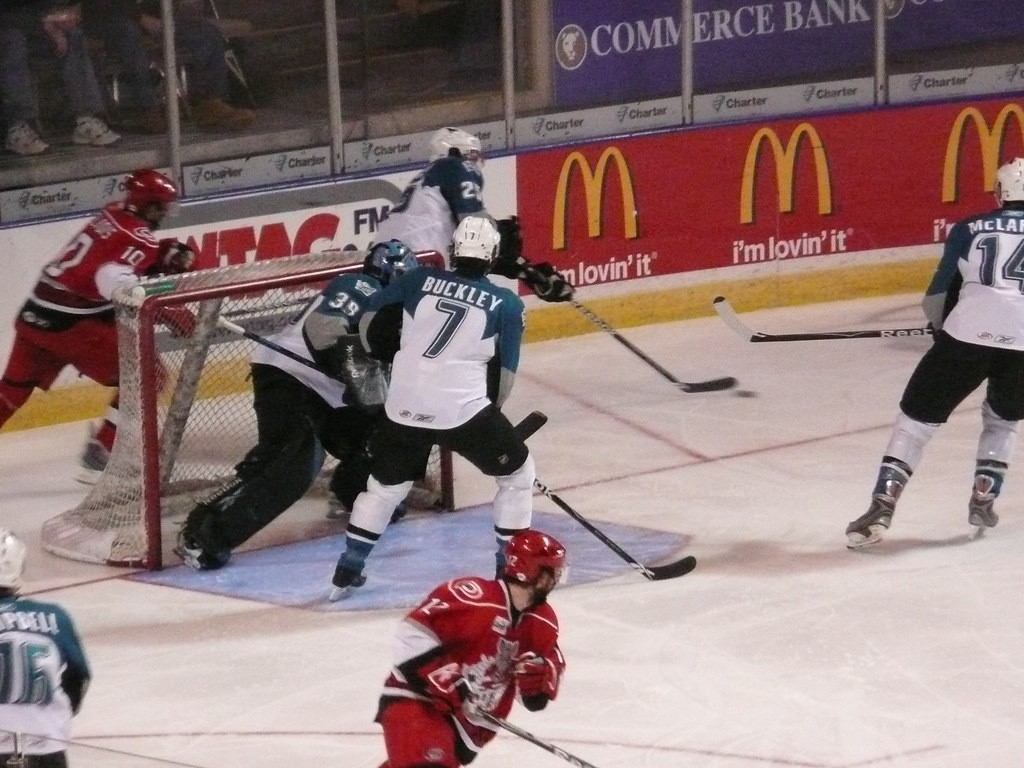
<box><xmin>0</xmin><ymin>167</ymin><xmax>197</xmax><ymax>486</ymax></box>
<box><xmin>367</xmin><ymin>127</ymin><xmax>577</xmax><ymax>273</ymax></box>
<box><xmin>844</xmin><ymin>158</ymin><xmax>1024</xmax><ymax>550</ymax></box>
<box><xmin>0</xmin><ymin>526</ymin><xmax>92</xmax><ymax>768</ymax></box>
<box><xmin>373</xmin><ymin>529</ymin><xmax>569</xmax><ymax>768</ymax></box>
<box><xmin>328</xmin><ymin>216</ymin><xmax>535</xmax><ymax>603</ymax></box>
<box><xmin>0</xmin><ymin>0</ymin><xmax>253</xmax><ymax>154</ymax></box>
<box><xmin>172</xmin><ymin>238</ymin><xmax>420</xmax><ymax>573</ymax></box>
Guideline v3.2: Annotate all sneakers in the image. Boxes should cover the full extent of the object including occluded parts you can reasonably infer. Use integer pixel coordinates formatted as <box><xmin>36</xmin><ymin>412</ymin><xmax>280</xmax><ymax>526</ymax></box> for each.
<box><xmin>171</xmin><ymin>527</ymin><xmax>225</xmax><ymax>571</ymax></box>
<box><xmin>844</xmin><ymin>479</ymin><xmax>904</xmax><ymax>550</ymax></box>
<box><xmin>72</xmin><ymin>116</ymin><xmax>121</xmax><ymax>146</ymax></box>
<box><xmin>75</xmin><ymin>416</ymin><xmax>118</xmax><ymax>484</ymax></box>
<box><xmin>5</xmin><ymin>124</ymin><xmax>50</xmax><ymax>155</ymax></box>
<box><xmin>495</xmin><ymin>552</ymin><xmax>507</xmax><ymax>580</ymax></box>
<box><xmin>329</xmin><ymin>554</ymin><xmax>367</xmax><ymax>602</ymax></box>
<box><xmin>327</xmin><ymin>490</ymin><xmax>408</xmax><ymax>527</ymax></box>
<box><xmin>966</xmin><ymin>473</ymin><xmax>999</xmax><ymax>541</ymax></box>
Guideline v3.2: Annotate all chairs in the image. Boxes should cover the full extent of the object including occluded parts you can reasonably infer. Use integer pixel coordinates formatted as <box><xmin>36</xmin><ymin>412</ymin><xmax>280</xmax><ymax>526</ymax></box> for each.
<box><xmin>100</xmin><ymin>0</ymin><xmax>253</xmax><ymax>117</ymax></box>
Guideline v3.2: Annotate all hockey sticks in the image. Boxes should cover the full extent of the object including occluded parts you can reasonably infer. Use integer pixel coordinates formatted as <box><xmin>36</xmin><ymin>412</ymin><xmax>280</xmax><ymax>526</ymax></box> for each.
<box><xmin>214</xmin><ymin>314</ymin><xmax>549</xmax><ymax>444</ymax></box>
<box><xmin>516</xmin><ymin>256</ymin><xmax>740</xmax><ymax>394</ymax></box>
<box><xmin>532</xmin><ymin>477</ymin><xmax>697</xmax><ymax>581</ymax></box>
<box><xmin>474</xmin><ymin>708</ymin><xmax>596</xmax><ymax>768</ymax></box>
<box><xmin>712</xmin><ymin>296</ymin><xmax>933</xmax><ymax>343</ymax></box>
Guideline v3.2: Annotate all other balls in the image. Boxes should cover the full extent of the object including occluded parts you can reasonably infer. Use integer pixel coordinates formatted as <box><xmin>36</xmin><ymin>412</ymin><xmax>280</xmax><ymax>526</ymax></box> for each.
<box><xmin>736</xmin><ymin>390</ymin><xmax>757</xmax><ymax>397</ymax></box>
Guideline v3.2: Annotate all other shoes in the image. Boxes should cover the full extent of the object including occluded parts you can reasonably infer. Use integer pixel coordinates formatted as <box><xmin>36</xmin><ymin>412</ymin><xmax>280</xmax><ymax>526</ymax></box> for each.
<box><xmin>121</xmin><ymin>103</ymin><xmax>168</xmax><ymax>136</ymax></box>
<box><xmin>195</xmin><ymin>98</ymin><xmax>256</xmax><ymax>132</ymax></box>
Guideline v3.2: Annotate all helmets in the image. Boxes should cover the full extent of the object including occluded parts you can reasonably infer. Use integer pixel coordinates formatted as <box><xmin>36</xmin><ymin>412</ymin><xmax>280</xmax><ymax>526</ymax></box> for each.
<box><xmin>452</xmin><ymin>216</ymin><xmax>496</xmax><ymax>265</ymax></box>
<box><xmin>994</xmin><ymin>156</ymin><xmax>1024</xmax><ymax>209</ymax></box>
<box><xmin>430</xmin><ymin>126</ymin><xmax>484</xmax><ymax>169</ymax></box>
<box><xmin>0</xmin><ymin>531</ymin><xmax>26</xmax><ymax>591</ymax></box>
<box><xmin>361</xmin><ymin>237</ymin><xmax>419</xmax><ymax>285</ymax></box>
<box><xmin>123</xmin><ymin>168</ymin><xmax>177</xmax><ymax>221</ymax></box>
<box><xmin>505</xmin><ymin>530</ymin><xmax>569</xmax><ymax>586</ymax></box>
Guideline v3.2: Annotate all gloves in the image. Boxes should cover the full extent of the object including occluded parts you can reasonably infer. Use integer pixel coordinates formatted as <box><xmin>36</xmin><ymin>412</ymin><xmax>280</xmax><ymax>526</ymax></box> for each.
<box><xmin>511</xmin><ymin>657</ymin><xmax>549</xmax><ymax>695</ymax></box>
<box><xmin>497</xmin><ymin>214</ymin><xmax>524</xmax><ymax>279</ymax></box>
<box><xmin>156</xmin><ymin>237</ymin><xmax>198</xmax><ymax>273</ymax></box>
<box><xmin>160</xmin><ymin>305</ymin><xmax>197</xmax><ymax>338</ymax></box>
<box><xmin>419</xmin><ymin>655</ymin><xmax>470</xmax><ymax>712</ymax></box>
<box><xmin>526</xmin><ymin>261</ymin><xmax>576</xmax><ymax>302</ymax></box>
